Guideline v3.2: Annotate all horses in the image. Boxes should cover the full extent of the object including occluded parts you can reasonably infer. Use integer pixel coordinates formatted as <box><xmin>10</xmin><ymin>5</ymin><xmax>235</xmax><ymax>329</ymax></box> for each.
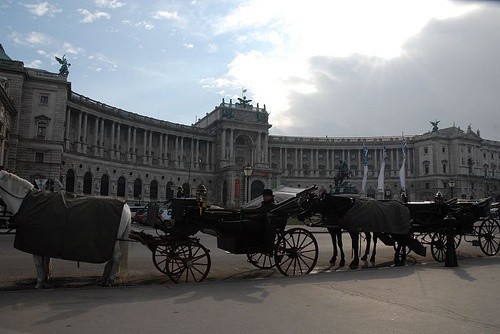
<box><xmin>297</xmin><ymin>189</ymin><xmax>410</xmax><ymax>269</ymax></box>
<box><xmin>0</xmin><ymin>170</ymin><xmax>132</xmax><ymax>289</ymax></box>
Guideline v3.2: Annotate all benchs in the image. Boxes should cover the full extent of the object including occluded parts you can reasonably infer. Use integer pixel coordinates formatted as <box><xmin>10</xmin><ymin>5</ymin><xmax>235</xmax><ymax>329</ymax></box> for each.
<box><xmin>406</xmin><ymin>200</ymin><xmax>449</xmax><ymax>217</ymax></box>
<box><xmin>455</xmin><ymin>198</ymin><xmax>489</xmax><ymax>208</ymax></box>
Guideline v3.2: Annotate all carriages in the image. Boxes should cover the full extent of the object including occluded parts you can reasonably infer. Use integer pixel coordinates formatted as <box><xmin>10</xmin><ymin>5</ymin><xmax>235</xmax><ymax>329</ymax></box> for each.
<box><xmin>291</xmin><ymin>183</ymin><xmax>500</xmax><ymax>270</ymax></box>
<box><xmin>0</xmin><ymin>165</ymin><xmax>319</xmax><ymax>292</ymax></box>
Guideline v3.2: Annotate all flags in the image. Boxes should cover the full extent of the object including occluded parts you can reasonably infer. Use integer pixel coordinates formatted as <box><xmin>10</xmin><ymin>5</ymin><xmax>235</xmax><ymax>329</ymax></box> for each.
<box><xmin>362</xmin><ymin>141</ymin><xmax>406</xmax><ymax>192</ymax></box>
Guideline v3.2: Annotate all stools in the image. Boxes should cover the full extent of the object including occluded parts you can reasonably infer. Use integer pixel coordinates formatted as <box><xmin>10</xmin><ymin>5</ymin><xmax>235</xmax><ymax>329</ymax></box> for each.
<box><xmin>169</xmin><ymin>197</ymin><xmax>198</xmax><ymax>227</ymax></box>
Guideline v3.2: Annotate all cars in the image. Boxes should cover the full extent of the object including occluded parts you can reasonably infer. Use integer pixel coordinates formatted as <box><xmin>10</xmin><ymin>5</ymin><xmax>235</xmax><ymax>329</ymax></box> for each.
<box><xmin>129</xmin><ymin>207</ymin><xmax>175</xmax><ymax>227</ymax></box>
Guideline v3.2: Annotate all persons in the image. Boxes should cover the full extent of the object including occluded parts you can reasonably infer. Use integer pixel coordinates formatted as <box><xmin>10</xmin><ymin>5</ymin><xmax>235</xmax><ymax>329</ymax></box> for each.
<box><xmin>59</xmin><ymin>55</ymin><xmax>69</xmax><ymax>75</ymax></box>
<box><xmin>244</xmin><ymin>189</ymin><xmax>275</xmax><ymax>215</ymax></box>
<box><xmin>338</xmin><ymin>159</ymin><xmax>343</xmax><ymax>177</ymax></box>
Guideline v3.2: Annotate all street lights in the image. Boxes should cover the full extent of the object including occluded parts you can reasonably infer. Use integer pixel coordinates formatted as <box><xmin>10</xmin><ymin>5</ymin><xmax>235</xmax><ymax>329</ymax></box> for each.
<box><xmin>243</xmin><ymin>162</ymin><xmax>254</xmax><ymax>203</ymax></box>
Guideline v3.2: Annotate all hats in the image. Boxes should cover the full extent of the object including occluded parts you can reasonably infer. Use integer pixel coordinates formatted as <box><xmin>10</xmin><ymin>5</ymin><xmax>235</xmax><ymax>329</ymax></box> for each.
<box><xmin>261</xmin><ymin>189</ymin><xmax>275</xmax><ymax>197</ymax></box>
